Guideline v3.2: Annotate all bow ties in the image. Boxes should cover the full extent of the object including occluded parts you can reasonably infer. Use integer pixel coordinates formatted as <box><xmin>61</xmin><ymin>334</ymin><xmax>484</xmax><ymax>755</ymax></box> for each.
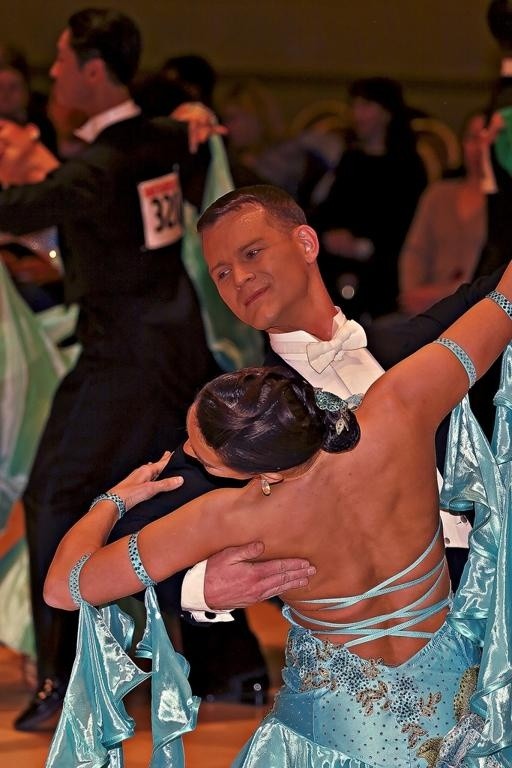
<box><xmin>306</xmin><ymin>319</ymin><xmax>368</xmax><ymax>374</ymax></box>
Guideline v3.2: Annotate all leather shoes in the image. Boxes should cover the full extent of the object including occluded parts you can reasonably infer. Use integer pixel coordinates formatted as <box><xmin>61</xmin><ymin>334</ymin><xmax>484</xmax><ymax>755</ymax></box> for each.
<box><xmin>15</xmin><ymin>677</ymin><xmax>69</xmax><ymax>729</ymax></box>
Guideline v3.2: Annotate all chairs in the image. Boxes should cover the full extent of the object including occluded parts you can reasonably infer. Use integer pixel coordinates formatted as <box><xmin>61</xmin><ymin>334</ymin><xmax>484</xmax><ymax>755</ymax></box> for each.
<box><xmin>214</xmin><ymin>78</ymin><xmax>462</xmax><ymax>183</ymax></box>
